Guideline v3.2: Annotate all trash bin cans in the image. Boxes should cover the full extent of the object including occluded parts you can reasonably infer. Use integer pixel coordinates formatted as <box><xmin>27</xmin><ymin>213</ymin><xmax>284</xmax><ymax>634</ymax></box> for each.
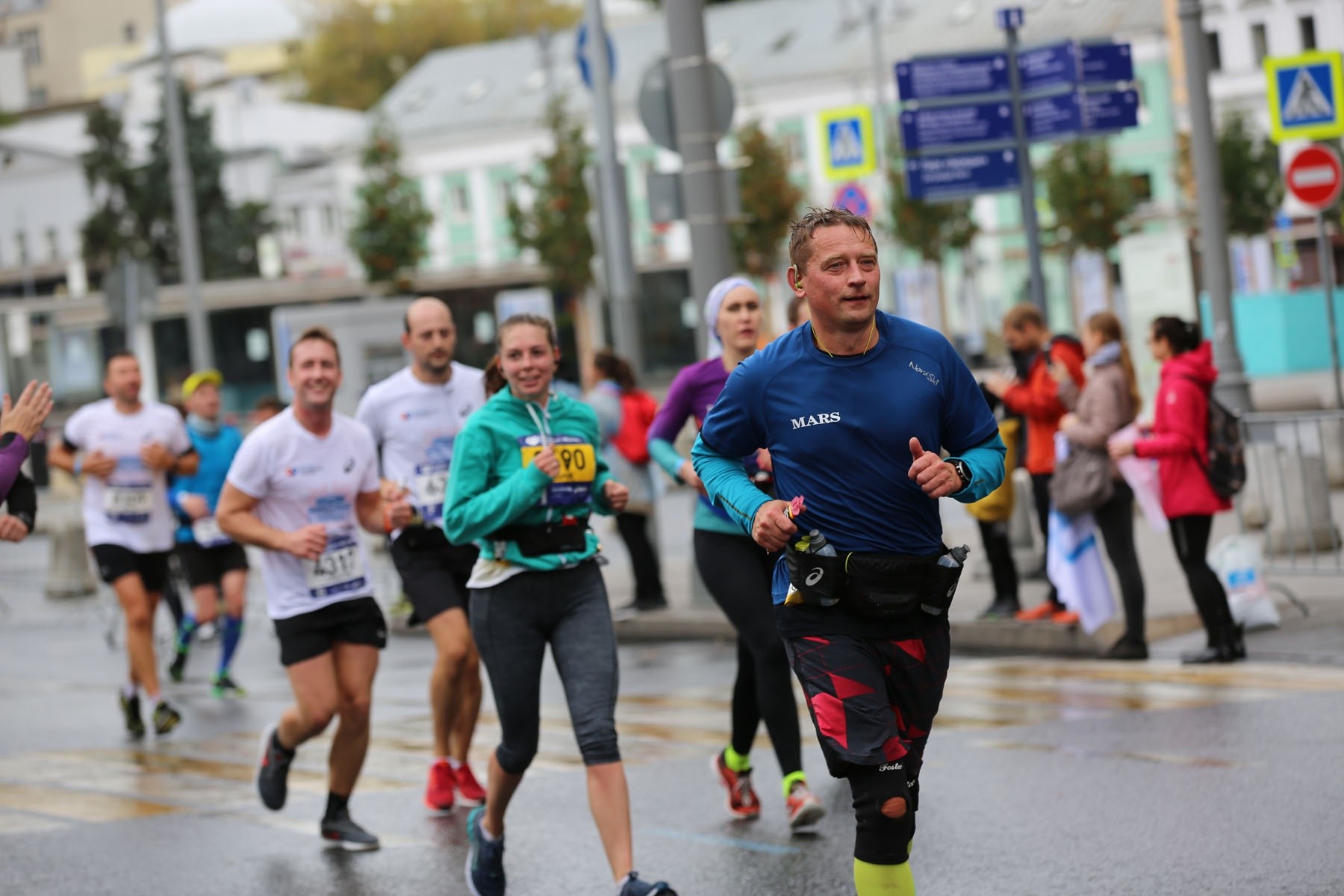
<box><xmin>1250</xmin><ymin>400</ymin><xmax>1343</xmax><ymax>556</ymax></box>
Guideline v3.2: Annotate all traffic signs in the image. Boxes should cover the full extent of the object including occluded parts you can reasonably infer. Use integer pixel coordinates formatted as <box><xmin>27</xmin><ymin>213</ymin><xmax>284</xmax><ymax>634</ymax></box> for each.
<box><xmin>1072</xmin><ymin>41</ymin><xmax>1132</xmax><ymax>84</ymax></box>
<box><xmin>1011</xmin><ymin>89</ymin><xmax>1082</xmax><ymax>141</ymax></box>
<box><xmin>898</xmin><ymin>100</ymin><xmax>1018</xmax><ymax>151</ymax></box>
<box><xmin>1009</xmin><ymin>35</ymin><xmax>1078</xmax><ymax>93</ymax></box>
<box><xmin>904</xmin><ymin>142</ymin><xmax>1021</xmax><ymax>200</ymax></box>
<box><xmin>895</xmin><ymin>51</ymin><xmax>1013</xmax><ymax>103</ymax></box>
<box><xmin>1081</xmin><ymin>87</ymin><xmax>1142</xmax><ymax>132</ymax></box>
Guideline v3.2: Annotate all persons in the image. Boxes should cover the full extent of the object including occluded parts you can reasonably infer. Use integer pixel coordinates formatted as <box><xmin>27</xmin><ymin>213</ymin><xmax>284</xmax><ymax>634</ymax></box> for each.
<box><xmin>46</xmin><ymin>350</ymin><xmax>199</xmax><ymax>735</ymax></box>
<box><xmin>443</xmin><ymin>317</ymin><xmax>679</xmax><ymax>896</ymax></box>
<box><xmin>214</xmin><ymin>331</ymin><xmax>413</xmax><ymax>851</ymax></box>
<box><xmin>1057</xmin><ymin>310</ymin><xmax>1151</xmax><ymax>660</ymax></box>
<box><xmin>646</xmin><ymin>276</ymin><xmax>826</xmax><ymax>829</ymax></box>
<box><xmin>983</xmin><ymin>304</ymin><xmax>1085</xmax><ymax>626</ymax></box>
<box><xmin>357</xmin><ymin>295</ymin><xmax>491</xmax><ymax>813</ymax></box>
<box><xmin>0</xmin><ymin>379</ymin><xmax>54</xmax><ymax>543</ymax></box>
<box><xmin>584</xmin><ymin>352</ymin><xmax>667</xmax><ymax>613</ymax></box>
<box><xmin>1107</xmin><ymin>316</ymin><xmax>1249</xmax><ymax>663</ymax></box>
<box><xmin>959</xmin><ymin>304</ymin><xmax>1024</xmax><ymax>623</ymax></box>
<box><xmin>691</xmin><ymin>208</ymin><xmax>1006</xmax><ymax>896</ymax></box>
<box><xmin>164</xmin><ymin>370</ymin><xmax>252</xmax><ymax>698</ymax></box>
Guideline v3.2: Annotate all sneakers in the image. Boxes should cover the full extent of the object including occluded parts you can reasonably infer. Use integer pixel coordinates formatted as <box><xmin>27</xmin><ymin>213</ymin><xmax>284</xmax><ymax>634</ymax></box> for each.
<box><xmin>464</xmin><ymin>807</ymin><xmax>506</xmax><ymax>896</ymax></box>
<box><xmin>785</xmin><ymin>779</ymin><xmax>825</xmax><ymax>827</ymax></box>
<box><xmin>711</xmin><ymin>746</ymin><xmax>760</xmax><ymax>819</ymax></box>
<box><xmin>451</xmin><ymin>763</ymin><xmax>488</xmax><ymax>802</ymax></box>
<box><xmin>424</xmin><ymin>761</ymin><xmax>455</xmax><ymax>809</ymax></box>
<box><xmin>619</xmin><ymin>871</ymin><xmax>678</xmax><ymax>896</ymax></box>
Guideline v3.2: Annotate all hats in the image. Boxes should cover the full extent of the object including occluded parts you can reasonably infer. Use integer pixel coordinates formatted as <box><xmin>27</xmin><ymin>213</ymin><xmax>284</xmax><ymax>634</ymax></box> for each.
<box><xmin>702</xmin><ymin>275</ymin><xmax>760</xmax><ymax>329</ymax></box>
<box><xmin>180</xmin><ymin>370</ymin><xmax>224</xmax><ymax>397</ymax></box>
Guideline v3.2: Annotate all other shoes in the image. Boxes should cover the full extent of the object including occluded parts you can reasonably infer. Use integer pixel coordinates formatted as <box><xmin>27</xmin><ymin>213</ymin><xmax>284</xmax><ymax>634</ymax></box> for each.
<box><xmin>1180</xmin><ymin>636</ymin><xmax>1246</xmax><ymax>663</ymax></box>
<box><xmin>256</xmin><ymin>728</ymin><xmax>295</xmax><ymax>809</ymax></box>
<box><xmin>167</xmin><ymin>633</ymin><xmax>189</xmax><ymax>680</ymax></box>
<box><xmin>1016</xmin><ymin>600</ymin><xmax>1080</xmax><ymax>626</ymax></box>
<box><xmin>977</xmin><ymin>599</ymin><xmax>1020</xmax><ymax>624</ymax></box>
<box><xmin>153</xmin><ymin>702</ymin><xmax>180</xmax><ymax>736</ymax></box>
<box><xmin>211</xmin><ymin>675</ymin><xmax>242</xmax><ymax>694</ymax></box>
<box><xmin>321</xmin><ymin>807</ymin><xmax>380</xmax><ymax>850</ymax></box>
<box><xmin>179</xmin><ymin>615</ymin><xmax>194</xmax><ymax>634</ymax></box>
<box><xmin>1103</xmin><ymin>632</ymin><xmax>1149</xmax><ymax>660</ymax></box>
<box><xmin>118</xmin><ymin>692</ymin><xmax>146</xmax><ymax>738</ymax></box>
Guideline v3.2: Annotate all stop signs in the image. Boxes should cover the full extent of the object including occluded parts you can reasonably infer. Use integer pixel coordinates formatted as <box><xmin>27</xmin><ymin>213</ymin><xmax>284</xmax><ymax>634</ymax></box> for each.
<box><xmin>1287</xmin><ymin>146</ymin><xmax>1341</xmax><ymax>204</ymax></box>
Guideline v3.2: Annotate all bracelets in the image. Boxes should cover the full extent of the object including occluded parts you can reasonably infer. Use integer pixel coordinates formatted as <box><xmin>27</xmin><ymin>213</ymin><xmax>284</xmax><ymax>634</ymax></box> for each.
<box><xmin>384</xmin><ymin>505</ymin><xmax>393</xmax><ymax>534</ymax></box>
<box><xmin>73</xmin><ymin>458</ymin><xmax>82</xmax><ymax>476</ymax></box>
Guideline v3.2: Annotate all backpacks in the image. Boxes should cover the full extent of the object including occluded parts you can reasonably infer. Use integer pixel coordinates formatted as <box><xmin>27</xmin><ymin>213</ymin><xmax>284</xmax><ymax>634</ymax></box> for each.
<box><xmin>1183</xmin><ymin>374</ymin><xmax>1246</xmax><ymax>496</ymax></box>
<box><xmin>603</xmin><ymin>390</ymin><xmax>659</xmax><ymax>465</ymax></box>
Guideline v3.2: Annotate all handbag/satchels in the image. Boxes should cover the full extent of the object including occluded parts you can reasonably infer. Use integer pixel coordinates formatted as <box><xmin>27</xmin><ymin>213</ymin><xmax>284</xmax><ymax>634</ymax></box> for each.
<box><xmin>785</xmin><ymin>541</ymin><xmax>964</xmax><ymax>643</ymax></box>
<box><xmin>481</xmin><ymin>519</ymin><xmax>591</xmax><ymax>557</ymax></box>
<box><xmin>1048</xmin><ymin>446</ymin><xmax>1115</xmax><ymax>516</ymax></box>
<box><xmin>1204</xmin><ymin>532</ymin><xmax>1283</xmax><ymax>631</ymax></box>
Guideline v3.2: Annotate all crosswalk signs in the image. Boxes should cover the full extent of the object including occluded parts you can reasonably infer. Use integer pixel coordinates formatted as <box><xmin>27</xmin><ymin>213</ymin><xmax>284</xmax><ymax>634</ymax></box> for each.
<box><xmin>1260</xmin><ymin>49</ymin><xmax>1344</xmax><ymax>145</ymax></box>
<box><xmin>818</xmin><ymin>106</ymin><xmax>877</xmax><ymax>181</ymax></box>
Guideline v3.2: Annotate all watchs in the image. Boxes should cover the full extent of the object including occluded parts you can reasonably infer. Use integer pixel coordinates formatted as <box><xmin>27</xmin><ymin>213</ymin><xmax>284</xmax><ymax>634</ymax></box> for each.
<box><xmin>948</xmin><ymin>458</ymin><xmax>971</xmax><ymax>489</ymax></box>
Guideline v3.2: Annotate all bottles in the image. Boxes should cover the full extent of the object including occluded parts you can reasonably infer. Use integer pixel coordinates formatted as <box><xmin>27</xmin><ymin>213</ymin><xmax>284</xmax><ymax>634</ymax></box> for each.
<box><xmin>806</xmin><ymin>529</ymin><xmax>840</xmax><ymax>607</ymax></box>
<box><xmin>921</xmin><ymin>544</ymin><xmax>971</xmax><ymax>616</ymax></box>
